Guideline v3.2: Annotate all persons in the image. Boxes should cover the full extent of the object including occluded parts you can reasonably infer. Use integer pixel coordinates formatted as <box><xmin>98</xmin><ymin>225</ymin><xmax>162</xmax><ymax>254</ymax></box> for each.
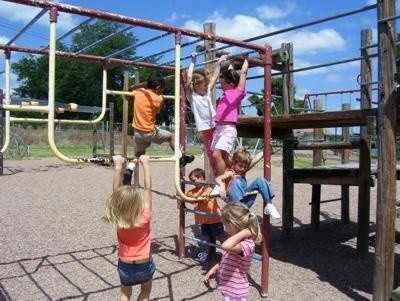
<box><xmin>185</xmin><ymin>52</ymin><xmax>229</xmax><ymax>175</ymax></box>
<box><xmin>168</xmin><ymin>167</ymin><xmax>230</xmax><ymax>264</ymax></box>
<box><xmin>200</xmin><ymin>201</ymin><xmax>264</xmax><ymax>301</ymax></box>
<box><xmin>214</xmin><ymin>138</ymin><xmax>280</xmax><ymax>220</ymax></box>
<box><xmin>100</xmin><ymin>155</ymin><xmax>155</xmax><ymax>301</ymax></box>
<box><xmin>122</xmin><ymin>72</ymin><xmax>196</xmax><ymax>177</ymax></box>
<box><xmin>208</xmin><ymin>48</ymin><xmax>249</xmax><ymax>197</ymax></box>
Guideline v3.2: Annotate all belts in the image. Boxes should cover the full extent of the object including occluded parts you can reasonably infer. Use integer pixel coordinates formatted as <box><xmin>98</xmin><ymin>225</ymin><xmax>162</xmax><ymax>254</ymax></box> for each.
<box><xmin>121</xmin><ymin>258</ymin><xmax>149</xmax><ymax>265</ymax></box>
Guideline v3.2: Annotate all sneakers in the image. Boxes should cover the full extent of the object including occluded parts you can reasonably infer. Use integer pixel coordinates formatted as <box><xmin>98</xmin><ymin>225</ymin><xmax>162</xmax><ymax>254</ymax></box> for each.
<box><xmin>123</xmin><ymin>169</ymin><xmax>133</xmax><ymax>186</ymax></box>
<box><xmin>264</xmin><ymin>202</ymin><xmax>281</xmax><ymax>220</ymax></box>
<box><xmin>210</xmin><ymin>184</ymin><xmax>221</xmax><ymax>196</ymax></box>
<box><xmin>199</xmin><ymin>253</ymin><xmax>210</xmax><ymax>263</ymax></box>
<box><xmin>179</xmin><ymin>155</ymin><xmax>195</xmax><ymax>168</ymax></box>
